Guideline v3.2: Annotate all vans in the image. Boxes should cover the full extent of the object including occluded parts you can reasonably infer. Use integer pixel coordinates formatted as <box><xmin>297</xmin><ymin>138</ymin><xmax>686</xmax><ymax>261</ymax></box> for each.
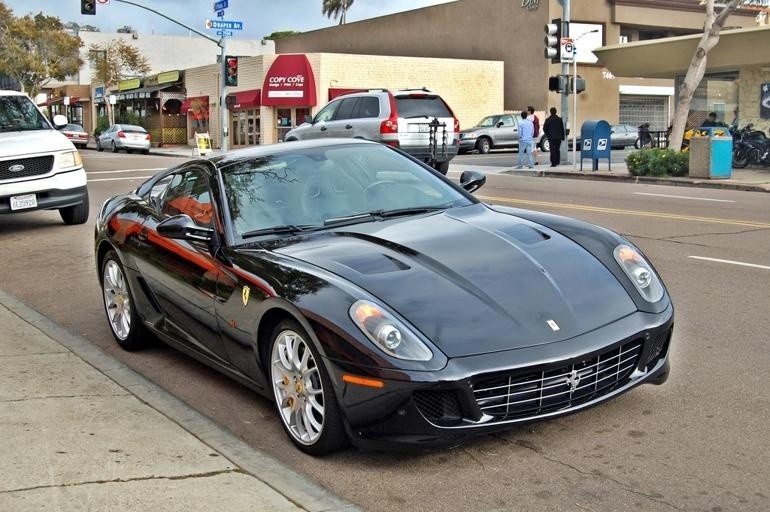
<box><xmin>0</xmin><ymin>88</ymin><xmax>90</xmax><ymax>230</ymax></box>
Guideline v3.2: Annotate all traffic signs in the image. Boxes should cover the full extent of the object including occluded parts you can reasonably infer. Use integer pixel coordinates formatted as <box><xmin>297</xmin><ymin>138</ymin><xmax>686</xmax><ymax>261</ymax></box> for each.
<box><xmin>216</xmin><ymin>11</ymin><xmax>224</xmax><ymax>17</ymax></box>
<box><xmin>204</xmin><ymin>20</ymin><xmax>243</xmax><ymax>31</ymax></box>
<box><xmin>213</xmin><ymin>1</ymin><xmax>228</xmax><ymax>10</ymax></box>
<box><xmin>217</xmin><ymin>31</ymin><xmax>232</xmax><ymax>37</ymax></box>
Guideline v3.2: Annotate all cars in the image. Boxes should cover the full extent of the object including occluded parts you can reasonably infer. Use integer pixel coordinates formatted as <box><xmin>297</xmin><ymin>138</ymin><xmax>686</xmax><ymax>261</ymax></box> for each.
<box><xmin>96</xmin><ymin>123</ymin><xmax>151</xmax><ymax>154</ymax></box>
<box><xmin>568</xmin><ymin>124</ymin><xmax>643</xmax><ymax>151</ymax></box>
<box><xmin>57</xmin><ymin>124</ymin><xmax>90</xmax><ymax>149</ymax></box>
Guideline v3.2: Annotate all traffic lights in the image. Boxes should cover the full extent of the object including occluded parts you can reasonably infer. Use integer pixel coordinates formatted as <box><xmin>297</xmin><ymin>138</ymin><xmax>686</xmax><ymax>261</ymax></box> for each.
<box><xmin>226</xmin><ymin>96</ymin><xmax>237</xmax><ymax>109</ymax></box>
<box><xmin>541</xmin><ymin>19</ymin><xmax>560</xmax><ymax>64</ymax></box>
<box><xmin>81</xmin><ymin>1</ymin><xmax>96</xmax><ymax>16</ymax></box>
<box><xmin>547</xmin><ymin>76</ymin><xmax>564</xmax><ymax>92</ymax></box>
<box><xmin>225</xmin><ymin>56</ymin><xmax>238</xmax><ymax>87</ymax></box>
<box><xmin>571</xmin><ymin>77</ymin><xmax>585</xmax><ymax>93</ymax></box>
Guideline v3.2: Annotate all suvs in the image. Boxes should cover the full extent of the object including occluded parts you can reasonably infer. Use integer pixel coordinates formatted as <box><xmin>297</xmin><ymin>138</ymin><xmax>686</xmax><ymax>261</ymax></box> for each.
<box><xmin>458</xmin><ymin>112</ymin><xmax>554</xmax><ymax>154</ymax></box>
<box><xmin>281</xmin><ymin>87</ymin><xmax>462</xmax><ymax>177</ymax></box>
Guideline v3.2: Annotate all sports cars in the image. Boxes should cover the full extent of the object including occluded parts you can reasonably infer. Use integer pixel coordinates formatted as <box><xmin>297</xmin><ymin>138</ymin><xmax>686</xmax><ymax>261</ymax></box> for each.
<box><xmin>101</xmin><ymin>134</ymin><xmax>680</xmax><ymax>469</ymax></box>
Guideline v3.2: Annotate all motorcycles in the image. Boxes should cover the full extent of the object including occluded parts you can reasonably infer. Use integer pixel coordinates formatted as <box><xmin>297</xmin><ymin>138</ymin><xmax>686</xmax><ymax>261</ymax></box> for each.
<box><xmin>636</xmin><ymin>121</ymin><xmax>770</xmax><ymax>168</ymax></box>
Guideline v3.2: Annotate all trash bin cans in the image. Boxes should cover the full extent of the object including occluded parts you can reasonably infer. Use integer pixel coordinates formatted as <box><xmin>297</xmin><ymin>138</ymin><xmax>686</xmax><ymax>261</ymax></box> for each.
<box><xmin>689</xmin><ymin>127</ymin><xmax>733</xmax><ymax>179</ymax></box>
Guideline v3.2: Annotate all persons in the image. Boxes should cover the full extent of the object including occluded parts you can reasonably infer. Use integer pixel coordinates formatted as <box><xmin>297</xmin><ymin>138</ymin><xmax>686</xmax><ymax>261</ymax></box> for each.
<box><xmin>543</xmin><ymin>108</ymin><xmax>564</xmax><ymax>167</ymax></box>
<box><xmin>516</xmin><ymin>112</ymin><xmax>535</xmax><ymax>169</ymax></box>
<box><xmin>526</xmin><ymin>107</ymin><xmax>539</xmax><ymax>167</ymax></box>
<box><xmin>701</xmin><ymin>113</ymin><xmax>722</xmax><ymax>127</ymax></box>
